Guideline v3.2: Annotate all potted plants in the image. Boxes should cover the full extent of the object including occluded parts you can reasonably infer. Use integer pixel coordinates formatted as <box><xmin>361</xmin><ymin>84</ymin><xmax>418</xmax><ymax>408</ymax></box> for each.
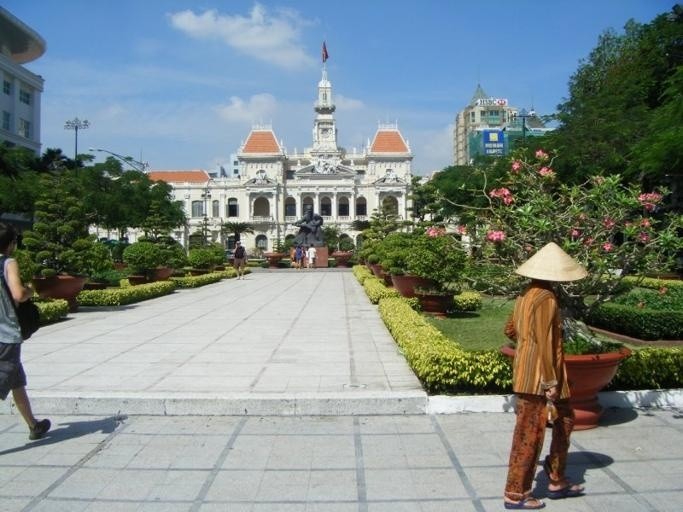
<box><xmin>428</xmin><ymin>147</ymin><xmax>675</xmax><ymax>430</ymax></box>
<box><xmin>20</xmin><ymin>172</ymin><xmax>256</xmax><ymax>314</ymax></box>
<box><xmin>330</xmin><ymin>224</ymin><xmax>483</xmax><ymax>319</ymax></box>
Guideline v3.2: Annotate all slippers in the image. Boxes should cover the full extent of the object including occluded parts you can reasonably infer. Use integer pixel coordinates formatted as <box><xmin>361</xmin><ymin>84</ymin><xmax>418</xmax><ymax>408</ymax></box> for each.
<box><xmin>502</xmin><ymin>495</ymin><xmax>547</xmax><ymax>510</ymax></box>
<box><xmin>545</xmin><ymin>480</ymin><xmax>586</xmax><ymax>499</ymax></box>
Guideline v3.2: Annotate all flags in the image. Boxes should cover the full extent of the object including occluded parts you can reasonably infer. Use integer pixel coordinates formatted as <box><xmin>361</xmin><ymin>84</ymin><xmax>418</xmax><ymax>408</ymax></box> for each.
<box><xmin>322</xmin><ymin>41</ymin><xmax>329</xmax><ymax>62</ymax></box>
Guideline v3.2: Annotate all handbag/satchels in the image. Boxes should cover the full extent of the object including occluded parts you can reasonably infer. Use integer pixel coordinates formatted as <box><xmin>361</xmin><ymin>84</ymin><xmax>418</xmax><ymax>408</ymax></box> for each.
<box><xmin>14</xmin><ymin>300</ymin><xmax>41</xmax><ymax>341</ymax></box>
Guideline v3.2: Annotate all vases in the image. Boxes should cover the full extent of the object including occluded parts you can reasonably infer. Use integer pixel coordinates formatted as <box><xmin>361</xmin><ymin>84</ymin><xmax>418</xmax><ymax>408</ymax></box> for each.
<box><xmin>263</xmin><ymin>252</ymin><xmax>285</xmax><ymax>269</ymax></box>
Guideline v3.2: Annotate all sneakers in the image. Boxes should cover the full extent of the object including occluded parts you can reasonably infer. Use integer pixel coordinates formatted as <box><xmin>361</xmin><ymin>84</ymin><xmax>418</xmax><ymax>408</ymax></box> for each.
<box><xmin>27</xmin><ymin>417</ymin><xmax>51</xmax><ymax>441</ymax></box>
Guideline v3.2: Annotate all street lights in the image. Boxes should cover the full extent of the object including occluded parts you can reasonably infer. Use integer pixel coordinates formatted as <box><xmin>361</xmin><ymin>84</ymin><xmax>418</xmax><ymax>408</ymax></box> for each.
<box><xmin>511</xmin><ymin>109</ymin><xmax>537</xmax><ymax>140</ymax></box>
<box><xmin>64</xmin><ymin>116</ymin><xmax>89</xmax><ymax>175</ymax></box>
<box><xmin>201</xmin><ymin>186</ymin><xmax>212</xmax><ymax>243</ymax></box>
<box><xmin>89</xmin><ymin>147</ymin><xmax>148</xmax><ymax>176</ymax></box>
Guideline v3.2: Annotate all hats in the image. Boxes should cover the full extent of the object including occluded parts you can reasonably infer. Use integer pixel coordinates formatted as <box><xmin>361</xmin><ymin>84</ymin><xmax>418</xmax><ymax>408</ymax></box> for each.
<box><xmin>512</xmin><ymin>240</ymin><xmax>588</xmax><ymax>283</ymax></box>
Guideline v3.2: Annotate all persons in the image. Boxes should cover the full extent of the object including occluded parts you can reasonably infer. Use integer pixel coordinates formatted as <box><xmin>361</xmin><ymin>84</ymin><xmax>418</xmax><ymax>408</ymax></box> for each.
<box><xmin>0</xmin><ymin>222</ymin><xmax>51</xmax><ymax>440</ymax></box>
<box><xmin>231</xmin><ymin>240</ymin><xmax>248</xmax><ymax>280</ymax></box>
<box><xmin>291</xmin><ymin>244</ymin><xmax>317</xmax><ymax>269</ymax></box>
<box><xmin>505</xmin><ymin>240</ymin><xmax>589</xmax><ymax>509</ymax></box>
<box><xmin>292</xmin><ymin>205</ymin><xmax>322</xmax><ymax>241</ymax></box>
